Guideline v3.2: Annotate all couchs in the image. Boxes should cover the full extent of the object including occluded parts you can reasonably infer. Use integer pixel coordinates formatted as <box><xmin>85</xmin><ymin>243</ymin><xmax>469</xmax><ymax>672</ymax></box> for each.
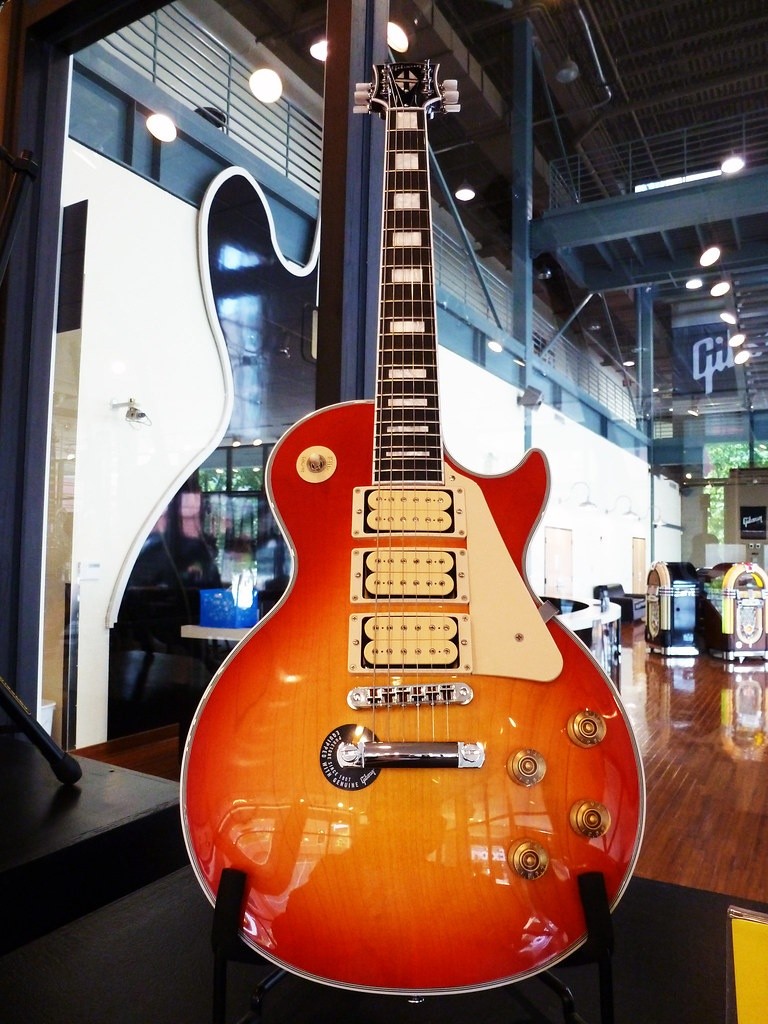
<box><xmin>594</xmin><ymin>584</ymin><xmax>646</xmax><ymax>625</ymax></box>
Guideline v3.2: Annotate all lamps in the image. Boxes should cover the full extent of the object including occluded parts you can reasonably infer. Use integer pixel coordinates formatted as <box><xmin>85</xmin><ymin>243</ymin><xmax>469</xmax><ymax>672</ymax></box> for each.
<box><xmin>387</xmin><ymin>17</ymin><xmax>418</xmax><ymax>57</ymax></box>
<box><xmin>452</xmin><ymin>178</ymin><xmax>478</xmax><ymax>204</ymax></box>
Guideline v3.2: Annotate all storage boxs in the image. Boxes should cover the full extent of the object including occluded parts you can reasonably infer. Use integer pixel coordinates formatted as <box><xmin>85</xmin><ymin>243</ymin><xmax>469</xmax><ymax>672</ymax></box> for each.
<box><xmin>200</xmin><ymin>589</ymin><xmax>258</xmax><ymax>628</ymax></box>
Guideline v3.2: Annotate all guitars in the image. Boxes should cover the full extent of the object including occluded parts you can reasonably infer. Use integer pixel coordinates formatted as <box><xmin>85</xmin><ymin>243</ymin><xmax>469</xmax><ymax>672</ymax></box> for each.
<box><xmin>176</xmin><ymin>53</ymin><xmax>649</xmax><ymax>995</ymax></box>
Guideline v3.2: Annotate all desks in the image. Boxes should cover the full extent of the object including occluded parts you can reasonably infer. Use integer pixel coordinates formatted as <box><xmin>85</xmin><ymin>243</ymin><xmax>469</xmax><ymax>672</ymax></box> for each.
<box><xmin>539</xmin><ymin>595</ymin><xmax>622</xmax><ymax>655</ymax></box>
